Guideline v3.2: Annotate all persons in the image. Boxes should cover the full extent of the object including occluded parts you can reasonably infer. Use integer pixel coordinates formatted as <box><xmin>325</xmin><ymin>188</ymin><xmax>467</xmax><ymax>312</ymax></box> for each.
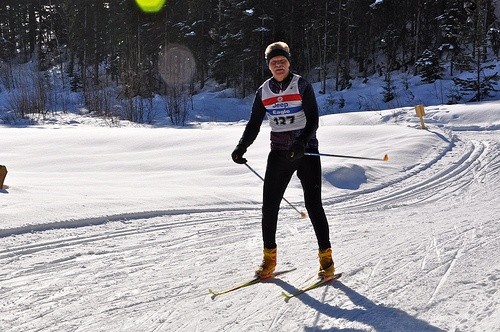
<box><xmin>231</xmin><ymin>42</ymin><xmax>334</xmax><ymax>276</ymax></box>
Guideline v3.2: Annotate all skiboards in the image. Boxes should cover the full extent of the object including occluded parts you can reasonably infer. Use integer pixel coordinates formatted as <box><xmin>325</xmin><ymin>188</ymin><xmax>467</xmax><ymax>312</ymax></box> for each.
<box><xmin>209</xmin><ymin>267</ymin><xmax>297</xmax><ymax>296</ymax></box>
<box><xmin>281</xmin><ymin>272</ymin><xmax>343</xmax><ymax>299</ymax></box>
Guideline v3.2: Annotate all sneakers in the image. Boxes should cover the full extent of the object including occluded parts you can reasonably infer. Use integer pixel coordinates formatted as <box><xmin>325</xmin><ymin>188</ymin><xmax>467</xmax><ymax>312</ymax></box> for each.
<box><xmin>317</xmin><ymin>247</ymin><xmax>335</xmax><ymax>277</ymax></box>
<box><xmin>255</xmin><ymin>247</ymin><xmax>277</xmax><ymax>276</ymax></box>
